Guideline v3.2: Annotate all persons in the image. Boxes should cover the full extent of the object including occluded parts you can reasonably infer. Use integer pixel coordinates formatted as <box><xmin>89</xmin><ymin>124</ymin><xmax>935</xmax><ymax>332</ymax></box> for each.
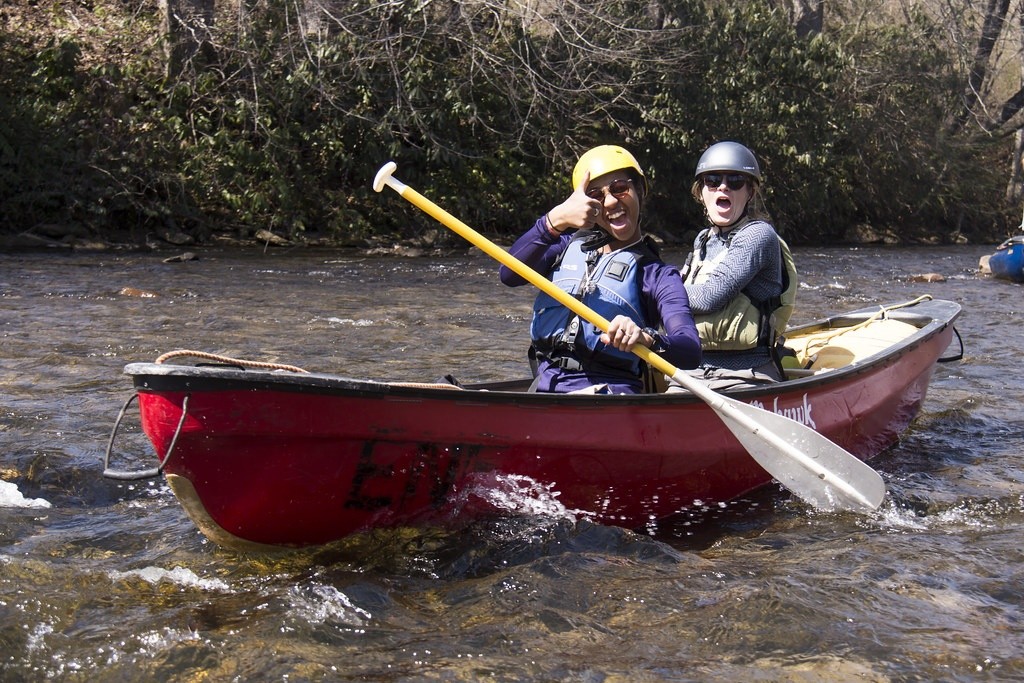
<box><xmin>497</xmin><ymin>144</ymin><xmax>705</xmax><ymax>392</ymax></box>
<box><xmin>665</xmin><ymin>142</ymin><xmax>800</xmax><ymax>394</ymax></box>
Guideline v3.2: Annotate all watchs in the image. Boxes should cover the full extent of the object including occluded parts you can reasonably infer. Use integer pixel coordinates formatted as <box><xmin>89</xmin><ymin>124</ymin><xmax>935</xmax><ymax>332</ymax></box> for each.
<box><xmin>640</xmin><ymin>327</ymin><xmax>661</xmax><ymax>353</ymax></box>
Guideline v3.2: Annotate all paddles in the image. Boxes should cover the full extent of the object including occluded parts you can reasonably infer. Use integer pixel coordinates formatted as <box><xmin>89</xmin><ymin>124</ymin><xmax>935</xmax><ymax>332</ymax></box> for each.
<box><xmin>371</xmin><ymin>161</ymin><xmax>885</xmax><ymax>514</ymax></box>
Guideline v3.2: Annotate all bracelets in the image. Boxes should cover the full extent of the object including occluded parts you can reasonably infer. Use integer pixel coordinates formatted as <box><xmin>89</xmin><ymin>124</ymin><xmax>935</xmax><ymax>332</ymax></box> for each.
<box><xmin>546</xmin><ymin>212</ymin><xmax>564</xmax><ymax>234</ymax></box>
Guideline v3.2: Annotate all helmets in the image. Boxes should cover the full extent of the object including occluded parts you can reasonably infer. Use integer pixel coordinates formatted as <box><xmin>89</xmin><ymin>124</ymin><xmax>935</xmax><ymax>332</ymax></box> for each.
<box><xmin>572</xmin><ymin>145</ymin><xmax>648</xmax><ymax>200</ymax></box>
<box><xmin>694</xmin><ymin>142</ymin><xmax>761</xmax><ymax>187</ymax></box>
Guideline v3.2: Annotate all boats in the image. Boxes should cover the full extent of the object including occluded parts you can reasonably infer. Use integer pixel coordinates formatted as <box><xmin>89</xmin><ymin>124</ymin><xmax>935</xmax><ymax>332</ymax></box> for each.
<box><xmin>103</xmin><ymin>292</ymin><xmax>963</xmax><ymax>561</ymax></box>
<box><xmin>978</xmin><ymin>237</ymin><xmax>1024</xmax><ymax>283</ymax></box>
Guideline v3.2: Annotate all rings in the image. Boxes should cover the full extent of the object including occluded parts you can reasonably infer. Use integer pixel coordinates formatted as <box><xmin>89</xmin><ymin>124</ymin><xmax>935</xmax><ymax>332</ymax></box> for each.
<box><xmin>593</xmin><ymin>208</ymin><xmax>600</xmax><ymax>217</ymax></box>
<box><xmin>625</xmin><ymin>333</ymin><xmax>632</xmax><ymax>338</ymax></box>
<box><xmin>617</xmin><ymin>328</ymin><xmax>625</xmax><ymax>335</ymax></box>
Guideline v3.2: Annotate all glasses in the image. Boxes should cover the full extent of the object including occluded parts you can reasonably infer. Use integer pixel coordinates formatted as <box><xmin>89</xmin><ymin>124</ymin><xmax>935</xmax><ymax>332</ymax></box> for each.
<box><xmin>701</xmin><ymin>171</ymin><xmax>747</xmax><ymax>192</ymax></box>
<box><xmin>585</xmin><ymin>178</ymin><xmax>635</xmax><ymax>205</ymax></box>
